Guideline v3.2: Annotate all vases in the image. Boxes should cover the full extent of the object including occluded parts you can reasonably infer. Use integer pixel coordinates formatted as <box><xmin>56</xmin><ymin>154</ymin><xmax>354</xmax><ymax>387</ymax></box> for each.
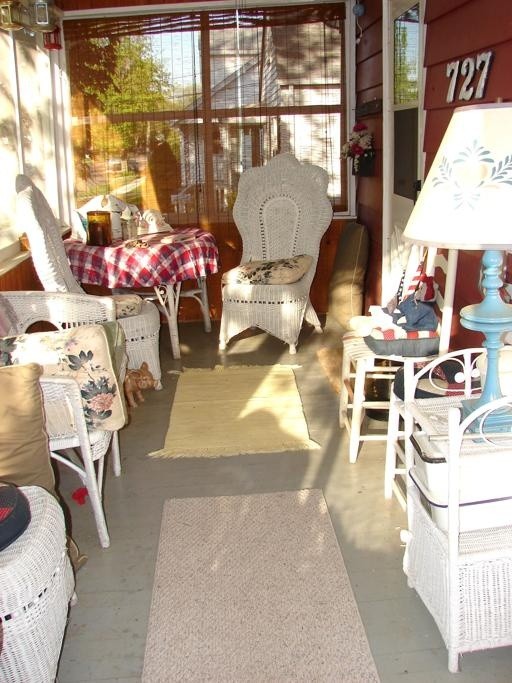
<box><xmin>350</xmin><ymin>156</ymin><xmax>372</xmax><ymax>176</ymax></box>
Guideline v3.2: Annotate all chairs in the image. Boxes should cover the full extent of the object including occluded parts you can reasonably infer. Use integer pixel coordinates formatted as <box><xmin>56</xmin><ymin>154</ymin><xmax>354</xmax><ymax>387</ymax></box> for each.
<box><xmin>220</xmin><ymin>150</ymin><xmax>335</xmax><ymax>354</ymax></box>
<box><xmin>335</xmin><ymin>236</ymin><xmax>460</xmax><ymax>463</ymax></box>
<box><xmin>0</xmin><ymin>288</ymin><xmax>127</xmax><ymax>545</ymax></box>
<box><xmin>13</xmin><ymin>170</ymin><xmax>166</xmax><ymax>391</ymax></box>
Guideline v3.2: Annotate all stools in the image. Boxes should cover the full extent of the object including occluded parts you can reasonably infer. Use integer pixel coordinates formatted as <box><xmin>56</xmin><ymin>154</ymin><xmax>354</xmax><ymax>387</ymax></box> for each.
<box><xmin>1</xmin><ymin>483</ymin><xmax>81</xmax><ymax>681</ymax></box>
<box><xmin>381</xmin><ymin>376</ymin><xmax>470</xmax><ymax>511</ymax></box>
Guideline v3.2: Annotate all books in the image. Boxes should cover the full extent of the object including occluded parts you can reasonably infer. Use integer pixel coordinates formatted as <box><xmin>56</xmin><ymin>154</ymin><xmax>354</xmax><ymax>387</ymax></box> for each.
<box><xmin>150</xmin><ymin>232</ymin><xmax>196</xmax><ymax>244</ymax></box>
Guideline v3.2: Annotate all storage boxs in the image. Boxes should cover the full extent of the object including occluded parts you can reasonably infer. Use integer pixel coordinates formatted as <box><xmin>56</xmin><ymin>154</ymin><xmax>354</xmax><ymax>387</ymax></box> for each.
<box><xmin>405</xmin><ymin>428</ymin><xmax>512</xmax><ymax>499</ymax></box>
<box><xmin>407</xmin><ymin>467</ymin><xmax>512</xmax><ymax>534</ymax></box>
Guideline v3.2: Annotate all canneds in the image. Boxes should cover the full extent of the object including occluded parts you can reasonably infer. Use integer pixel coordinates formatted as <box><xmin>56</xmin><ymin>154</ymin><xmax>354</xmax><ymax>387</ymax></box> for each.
<box><xmin>121</xmin><ymin>217</ymin><xmax>138</xmax><ymax>241</ymax></box>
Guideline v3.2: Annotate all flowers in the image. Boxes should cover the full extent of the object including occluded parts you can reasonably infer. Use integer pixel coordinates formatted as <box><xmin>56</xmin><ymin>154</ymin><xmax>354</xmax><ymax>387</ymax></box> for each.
<box><xmin>342</xmin><ymin>120</ymin><xmax>375</xmax><ymax>175</ymax></box>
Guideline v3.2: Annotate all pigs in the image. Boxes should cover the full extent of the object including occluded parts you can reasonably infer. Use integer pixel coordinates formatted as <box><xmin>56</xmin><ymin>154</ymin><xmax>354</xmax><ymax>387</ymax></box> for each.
<box><xmin>123</xmin><ymin>361</ymin><xmax>159</xmax><ymax>409</ymax></box>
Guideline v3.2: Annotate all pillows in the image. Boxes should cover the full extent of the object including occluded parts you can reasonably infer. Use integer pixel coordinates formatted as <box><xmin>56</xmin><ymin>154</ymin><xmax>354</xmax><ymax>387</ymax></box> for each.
<box><xmin>1</xmin><ymin>360</ymin><xmax>88</xmax><ymax>572</ymax></box>
<box><xmin>223</xmin><ymin>251</ymin><xmax>311</xmax><ymax>284</ymax></box>
<box><xmin>0</xmin><ymin>324</ymin><xmax>126</xmax><ymax>433</ymax></box>
<box><xmin>322</xmin><ymin>217</ymin><xmax>370</xmax><ymax>336</ymax></box>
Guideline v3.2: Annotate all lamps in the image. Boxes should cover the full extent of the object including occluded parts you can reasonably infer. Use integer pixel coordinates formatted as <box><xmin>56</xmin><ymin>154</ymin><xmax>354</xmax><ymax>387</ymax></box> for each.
<box><xmin>403</xmin><ymin>98</ymin><xmax>512</xmax><ymax>440</ymax></box>
<box><xmin>0</xmin><ymin>0</ymin><xmax>64</xmax><ymax>54</ymax></box>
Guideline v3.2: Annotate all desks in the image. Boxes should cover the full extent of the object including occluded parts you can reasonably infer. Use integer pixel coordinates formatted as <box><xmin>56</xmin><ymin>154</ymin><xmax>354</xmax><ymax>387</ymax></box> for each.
<box><xmin>66</xmin><ymin>225</ymin><xmax>217</xmax><ymax>359</ymax></box>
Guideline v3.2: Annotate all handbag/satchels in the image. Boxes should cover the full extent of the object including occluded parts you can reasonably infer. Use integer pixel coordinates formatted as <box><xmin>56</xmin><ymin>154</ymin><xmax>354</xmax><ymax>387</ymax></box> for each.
<box><xmin>392</xmin><ymin>294</ymin><xmax>438</xmax><ymax>330</ymax></box>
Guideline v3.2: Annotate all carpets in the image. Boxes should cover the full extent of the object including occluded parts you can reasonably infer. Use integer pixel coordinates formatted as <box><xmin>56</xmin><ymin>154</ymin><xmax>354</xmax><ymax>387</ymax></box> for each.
<box><xmin>140</xmin><ymin>485</ymin><xmax>384</xmax><ymax>681</ymax></box>
<box><xmin>147</xmin><ymin>363</ymin><xmax>328</xmax><ymax>456</ymax></box>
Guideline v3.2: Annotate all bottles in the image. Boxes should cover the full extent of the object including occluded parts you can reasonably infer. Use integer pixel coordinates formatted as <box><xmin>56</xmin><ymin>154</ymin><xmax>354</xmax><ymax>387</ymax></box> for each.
<box><xmin>121</xmin><ymin>219</ymin><xmax>137</xmax><ymax>239</ymax></box>
<box><xmin>86</xmin><ymin>210</ymin><xmax>111</xmax><ymax>244</ymax></box>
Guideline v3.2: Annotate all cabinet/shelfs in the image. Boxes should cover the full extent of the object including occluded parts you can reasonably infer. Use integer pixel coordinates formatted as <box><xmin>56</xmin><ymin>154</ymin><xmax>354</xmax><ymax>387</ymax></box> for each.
<box><xmin>391</xmin><ymin>340</ymin><xmax>512</xmax><ymax>672</ymax></box>
